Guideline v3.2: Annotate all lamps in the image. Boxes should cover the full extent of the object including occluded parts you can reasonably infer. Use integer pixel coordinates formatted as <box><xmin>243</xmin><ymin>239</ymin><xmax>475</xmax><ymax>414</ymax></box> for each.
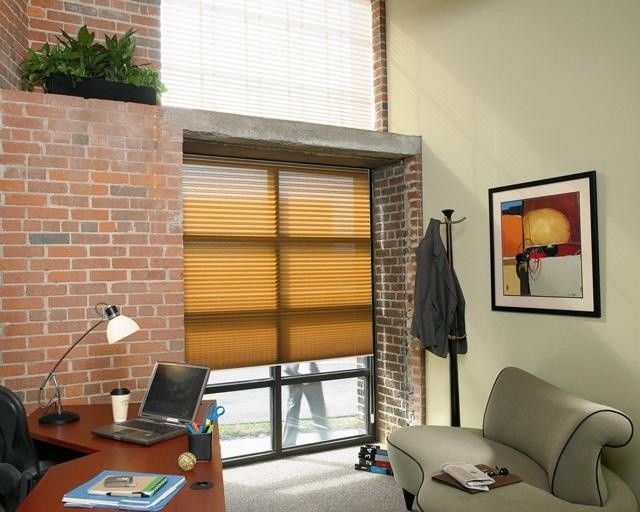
<box><xmin>35</xmin><ymin>302</ymin><xmax>142</xmax><ymax>425</ymax></box>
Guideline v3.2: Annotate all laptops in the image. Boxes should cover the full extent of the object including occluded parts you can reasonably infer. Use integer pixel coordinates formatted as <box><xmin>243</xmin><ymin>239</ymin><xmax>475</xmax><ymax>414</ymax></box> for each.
<box><xmin>89</xmin><ymin>360</ymin><xmax>211</xmax><ymax>446</ymax></box>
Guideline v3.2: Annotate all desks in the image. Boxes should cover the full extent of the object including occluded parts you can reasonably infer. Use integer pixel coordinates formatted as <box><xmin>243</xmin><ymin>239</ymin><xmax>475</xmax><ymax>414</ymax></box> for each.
<box><xmin>17</xmin><ymin>398</ymin><xmax>226</xmax><ymax>512</ymax></box>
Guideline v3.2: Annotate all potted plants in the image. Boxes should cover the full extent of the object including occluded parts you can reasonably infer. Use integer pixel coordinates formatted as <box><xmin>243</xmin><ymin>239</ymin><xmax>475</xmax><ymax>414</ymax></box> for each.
<box><xmin>20</xmin><ymin>23</ymin><xmax>167</xmax><ymax>106</ymax></box>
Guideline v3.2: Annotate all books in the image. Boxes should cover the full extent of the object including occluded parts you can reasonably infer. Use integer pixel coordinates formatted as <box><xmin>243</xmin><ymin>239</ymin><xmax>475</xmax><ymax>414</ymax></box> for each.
<box><xmin>62</xmin><ymin>469</ymin><xmax>188</xmax><ymax>512</ymax></box>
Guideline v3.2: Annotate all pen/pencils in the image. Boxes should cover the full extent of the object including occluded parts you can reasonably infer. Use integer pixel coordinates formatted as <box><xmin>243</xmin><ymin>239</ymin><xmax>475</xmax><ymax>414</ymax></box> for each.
<box><xmin>186</xmin><ymin>417</ymin><xmax>213</xmax><ymax>433</ymax></box>
<box><xmin>106</xmin><ymin>491</ymin><xmax>150</xmax><ymax>498</ymax></box>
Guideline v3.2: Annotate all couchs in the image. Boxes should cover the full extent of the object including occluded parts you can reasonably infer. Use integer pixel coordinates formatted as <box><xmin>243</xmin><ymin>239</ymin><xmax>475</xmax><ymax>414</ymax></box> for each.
<box><xmin>386</xmin><ymin>365</ymin><xmax>639</xmax><ymax>512</ymax></box>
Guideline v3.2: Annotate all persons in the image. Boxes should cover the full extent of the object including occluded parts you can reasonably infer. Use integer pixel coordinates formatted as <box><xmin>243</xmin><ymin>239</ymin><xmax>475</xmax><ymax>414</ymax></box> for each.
<box><xmin>282</xmin><ymin>362</ymin><xmax>329</xmax><ymax>448</ymax></box>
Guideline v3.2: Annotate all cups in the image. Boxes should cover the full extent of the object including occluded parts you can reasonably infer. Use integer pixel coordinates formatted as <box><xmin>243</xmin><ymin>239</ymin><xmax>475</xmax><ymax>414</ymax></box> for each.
<box><xmin>110</xmin><ymin>388</ymin><xmax>130</xmax><ymax>422</ymax></box>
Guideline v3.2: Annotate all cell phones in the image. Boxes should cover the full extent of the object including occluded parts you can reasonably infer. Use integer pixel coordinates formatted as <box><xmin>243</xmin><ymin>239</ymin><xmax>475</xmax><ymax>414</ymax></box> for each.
<box><xmin>104</xmin><ymin>476</ymin><xmax>136</xmax><ymax>487</ymax></box>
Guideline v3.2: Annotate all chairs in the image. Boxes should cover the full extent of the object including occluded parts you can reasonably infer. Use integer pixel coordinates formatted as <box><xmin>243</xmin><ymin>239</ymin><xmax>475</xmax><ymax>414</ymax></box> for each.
<box><xmin>1</xmin><ymin>384</ymin><xmax>48</xmax><ymax>512</ymax></box>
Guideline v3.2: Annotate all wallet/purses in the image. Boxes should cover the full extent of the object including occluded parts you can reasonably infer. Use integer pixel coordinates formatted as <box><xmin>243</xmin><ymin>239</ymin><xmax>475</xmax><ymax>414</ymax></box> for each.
<box><xmin>441</xmin><ymin>462</ymin><xmax>497</xmax><ymax>491</ymax></box>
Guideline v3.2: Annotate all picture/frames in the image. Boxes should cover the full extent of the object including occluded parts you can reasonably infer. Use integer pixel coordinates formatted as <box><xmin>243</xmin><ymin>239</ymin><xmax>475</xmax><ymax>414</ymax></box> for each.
<box><xmin>488</xmin><ymin>168</ymin><xmax>602</xmax><ymax>318</ymax></box>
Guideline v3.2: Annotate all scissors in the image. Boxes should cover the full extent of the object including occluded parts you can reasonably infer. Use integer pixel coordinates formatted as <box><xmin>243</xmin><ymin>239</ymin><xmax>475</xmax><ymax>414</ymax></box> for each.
<box><xmin>204</xmin><ymin>402</ymin><xmax>225</xmax><ymax>424</ymax></box>
